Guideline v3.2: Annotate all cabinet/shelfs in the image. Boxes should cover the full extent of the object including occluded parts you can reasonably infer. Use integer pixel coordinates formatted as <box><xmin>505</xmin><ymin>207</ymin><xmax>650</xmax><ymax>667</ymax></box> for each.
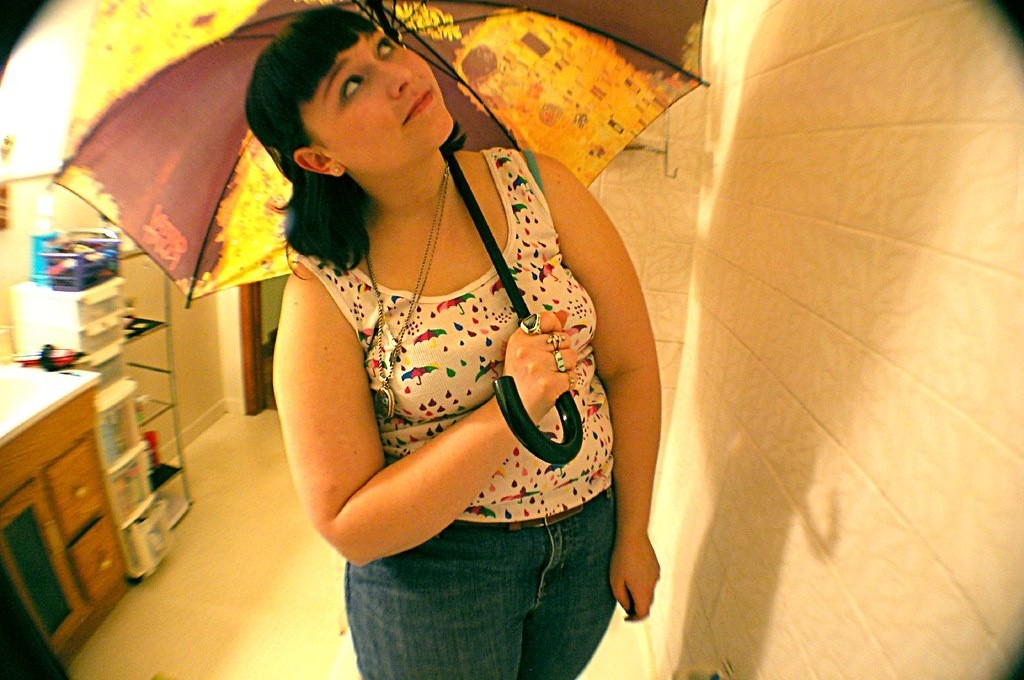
<box><xmin>0</xmin><ymin>250</ymin><xmax>192</xmax><ymax>680</ymax></box>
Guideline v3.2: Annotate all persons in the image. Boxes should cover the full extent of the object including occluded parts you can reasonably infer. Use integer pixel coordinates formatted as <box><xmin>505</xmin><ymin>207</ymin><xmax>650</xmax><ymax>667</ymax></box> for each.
<box><xmin>243</xmin><ymin>4</ymin><xmax>663</xmax><ymax>680</ymax></box>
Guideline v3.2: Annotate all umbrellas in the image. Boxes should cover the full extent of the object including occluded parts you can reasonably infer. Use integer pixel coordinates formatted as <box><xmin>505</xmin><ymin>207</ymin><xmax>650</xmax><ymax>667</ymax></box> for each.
<box><xmin>0</xmin><ymin>0</ymin><xmax>709</xmax><ymax>464</ymax></box>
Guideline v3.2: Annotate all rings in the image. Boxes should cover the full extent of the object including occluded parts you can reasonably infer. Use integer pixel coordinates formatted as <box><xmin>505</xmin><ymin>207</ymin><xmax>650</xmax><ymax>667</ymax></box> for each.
<box><xmin>546</xmin><ymin>330</ymin><xmax>566</xmax><ymax>351</ymax></box>
<box><xmin>521</xmin><ymin>313</ymin><xmax>543</xmax><ymax>336</ymax></box>
<box><xmin>565</xmin><ymin>370</ymin><xmax>576</xmax><ymax>390</ymax></box>
<box><xmin>552</xmin><ymin>349</ymin><xmax>566</xmax><ymax>372</ymax></box>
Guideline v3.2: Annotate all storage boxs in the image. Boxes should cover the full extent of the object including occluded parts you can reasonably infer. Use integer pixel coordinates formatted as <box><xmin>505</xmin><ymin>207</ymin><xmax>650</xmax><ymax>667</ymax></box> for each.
<box><xmin>39</xmin><ymin>238</ymin><xmax>121</xmax><ymax>291</ymax></box>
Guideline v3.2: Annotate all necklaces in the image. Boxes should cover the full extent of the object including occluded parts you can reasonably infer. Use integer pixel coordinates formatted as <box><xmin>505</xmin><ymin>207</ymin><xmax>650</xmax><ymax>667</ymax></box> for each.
<box><xmin>362</xmin><ymin>159</ymin><xmax>449</xmax><ymax>417</ymax></box>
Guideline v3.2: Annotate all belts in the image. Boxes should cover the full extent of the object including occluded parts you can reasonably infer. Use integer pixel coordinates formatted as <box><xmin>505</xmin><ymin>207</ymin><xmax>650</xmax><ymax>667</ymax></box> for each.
<box><xmin>479</xmin><ymin>501</ymin><xmax>586</xmax><ymax>531</ymax></box>
